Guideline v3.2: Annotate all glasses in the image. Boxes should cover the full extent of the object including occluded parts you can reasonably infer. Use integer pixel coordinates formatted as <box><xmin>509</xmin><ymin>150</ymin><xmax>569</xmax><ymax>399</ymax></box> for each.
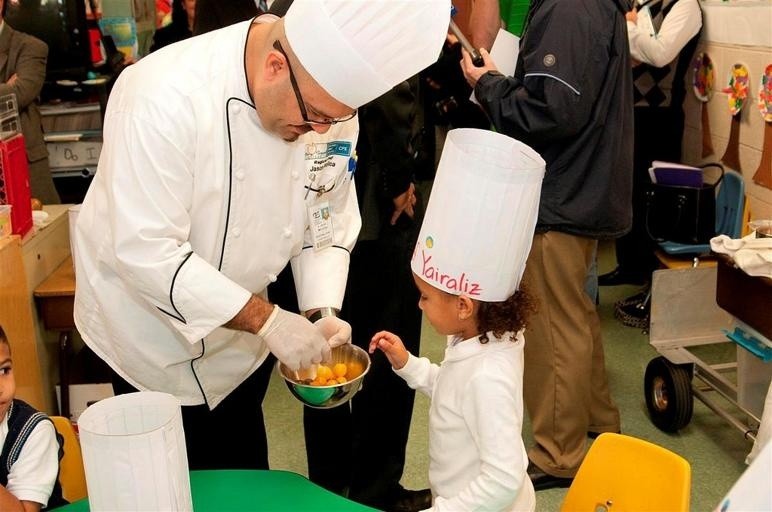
<box><xmin>272</xmin><ymin>39</ymin><xmax>356</xmax><ymax>126</ymax></box>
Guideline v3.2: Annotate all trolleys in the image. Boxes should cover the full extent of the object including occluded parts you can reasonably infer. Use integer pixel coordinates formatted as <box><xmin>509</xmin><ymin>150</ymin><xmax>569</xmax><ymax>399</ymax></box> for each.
<box><xmin>644</xmin><ymin>231</ymin><xmax>772</xmax><ymax>443</ymax></box>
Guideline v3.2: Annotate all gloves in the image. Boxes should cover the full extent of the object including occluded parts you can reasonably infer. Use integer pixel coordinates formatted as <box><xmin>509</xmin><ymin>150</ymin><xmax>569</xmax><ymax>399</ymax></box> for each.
<box><xmin>256</xmin><ymin>304</ymin><xmax>331</xmax><ymax>373</ymax></box>
<box><xmin>312</xmin><ymin>316</ymin><xmax>352</xmax><ymax>349</ymax></box>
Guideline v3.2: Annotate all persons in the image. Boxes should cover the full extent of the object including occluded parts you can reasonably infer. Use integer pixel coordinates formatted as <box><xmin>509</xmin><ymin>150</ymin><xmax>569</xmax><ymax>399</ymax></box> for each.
<box><xmin>598</xmin><ymin>0</ymin><xmax>704</xmax><ymax>285</ymax></box>
<box><xmin>459</xmin><ymin>0</ymin><xmax>633</xmax><ymax>490</ymax></box>
<box><xmin>369</xmin><ymin>128</ymin><xmax>536</xmax><ymax>511</ymax></box>
<box><xmin>72</xmin><ymin>1</ymin><xmax>451</xmax><ymax>471</ymax></box>
<box><xmin>303</xmin><ymin>74</ymin><xmax>432</xmax><ymax>511</ymax></box>
<box><xmin>1</xmin><ymin>328</ymin><xmax>70</xmax><ymax>512</ymax></box>
<box><xmin>1</xmin><ymin>2</ymin><xmax>259</xmax><ymax>206</ymax></box>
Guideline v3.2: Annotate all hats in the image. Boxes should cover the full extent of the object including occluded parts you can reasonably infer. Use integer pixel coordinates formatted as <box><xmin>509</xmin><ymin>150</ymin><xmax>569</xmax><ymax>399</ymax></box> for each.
<box><xmin>410</xmin><ymin>127</ymin><xmax>547</xmax><ymax>303</ymax></box>
<box><xmin>283</xmin><ymin>0</ymin><xmax>452</xmax><ymax>110</ymax></box>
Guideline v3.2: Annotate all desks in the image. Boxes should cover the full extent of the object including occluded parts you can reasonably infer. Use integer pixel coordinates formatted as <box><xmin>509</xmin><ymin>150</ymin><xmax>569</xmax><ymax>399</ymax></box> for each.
<box><xmin>33</xmin><ymin>252</ymin><xmax>76</xmax><ymax>420</ymax></box>
<box><xmin>41</xmin><ymin>469</ymin><xmax>387</xmax><ymax>512</ymax></box>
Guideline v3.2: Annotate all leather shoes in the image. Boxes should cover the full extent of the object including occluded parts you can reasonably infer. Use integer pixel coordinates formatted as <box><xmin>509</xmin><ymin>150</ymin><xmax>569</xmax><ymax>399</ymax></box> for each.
<box><xmin>595</xmin><ymin>264</ymin><xmax>645</xmax><ymax>286</ymax></box>
<box><xmin>527</xmin><ymin>460</ymin><xmax>573</xmax><ymax>492</ymax></box>
<box><xmin>386</xmin><ymin>489</ymin><xmax>432</xmax><ymax>512</ymax></box>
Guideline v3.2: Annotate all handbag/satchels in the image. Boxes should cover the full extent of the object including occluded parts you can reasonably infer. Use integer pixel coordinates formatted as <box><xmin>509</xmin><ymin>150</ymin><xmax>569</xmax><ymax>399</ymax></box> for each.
<box><xmin>637</xmin><ymin>162</ymin><xmax>725</xmax><ymax>245</ymax></box>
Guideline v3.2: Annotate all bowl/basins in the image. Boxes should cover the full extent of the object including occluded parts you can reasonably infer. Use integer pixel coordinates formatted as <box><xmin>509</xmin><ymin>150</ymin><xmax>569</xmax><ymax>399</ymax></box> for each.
<box><xmin>277</xmin><ymin>342</ymin><xmax>372</xmax><ymax>410</ymax></box>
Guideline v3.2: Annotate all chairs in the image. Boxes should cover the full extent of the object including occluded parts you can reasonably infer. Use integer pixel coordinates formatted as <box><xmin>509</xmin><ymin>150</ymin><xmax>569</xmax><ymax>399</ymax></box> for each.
<box><xmin>635</xmin><ymin>172</ymin><xmax>751</xmax><ymax>335</ymax></box>
<box><xmin>46</xmin><ymin>416</ymin><xmax>88</xmax><ymax>505</ymax></box>
<box><xmin>559</xmin><ymin>432</ymin><xmax>691</xmax><ymax>512</ymax></box>
